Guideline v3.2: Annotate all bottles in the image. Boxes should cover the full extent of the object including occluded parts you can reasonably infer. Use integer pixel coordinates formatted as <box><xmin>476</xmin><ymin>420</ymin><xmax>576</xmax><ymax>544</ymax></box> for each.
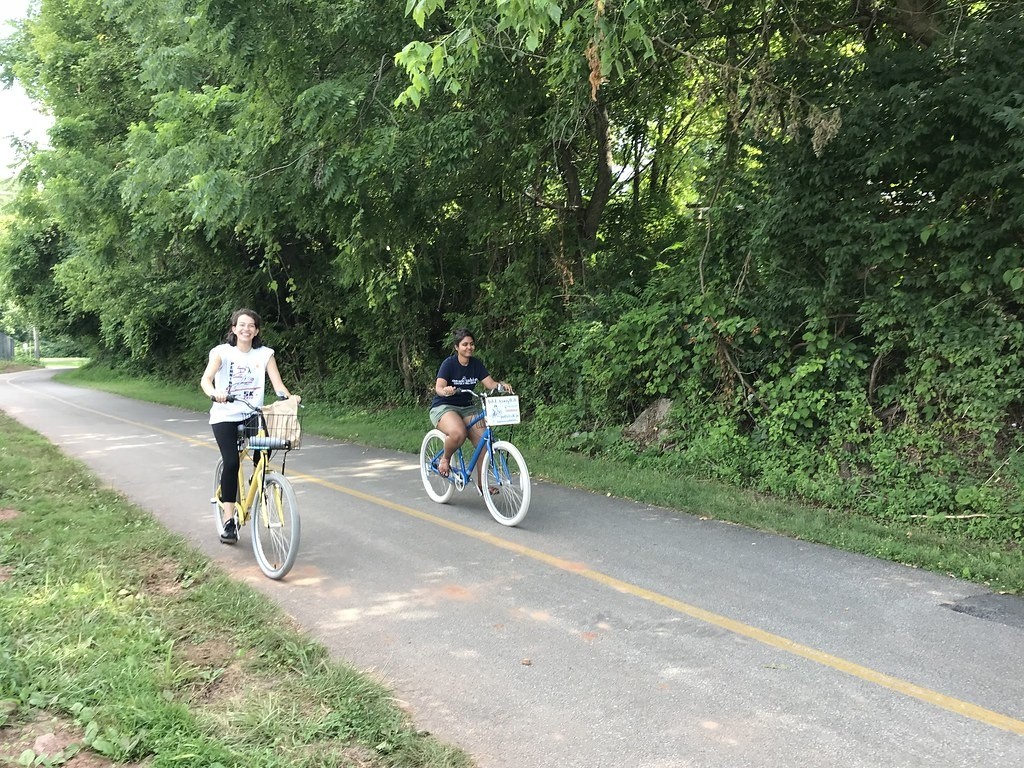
<box><xmin>249</xmin><ymin>436</ymin><xmax>291</xmax><ymax>449</ymax></box>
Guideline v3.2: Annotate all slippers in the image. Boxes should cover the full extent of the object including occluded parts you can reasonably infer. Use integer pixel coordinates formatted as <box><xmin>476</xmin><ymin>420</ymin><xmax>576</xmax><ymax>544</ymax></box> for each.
<box><xmin>438</xmin><ymin>456</ymin><xmax>450</xmax><ymax>478</ymax></box>
<box><xmin>478</xmin><ymin>486</ymin><xmax>499</xmax><ymax>496</ymax></box>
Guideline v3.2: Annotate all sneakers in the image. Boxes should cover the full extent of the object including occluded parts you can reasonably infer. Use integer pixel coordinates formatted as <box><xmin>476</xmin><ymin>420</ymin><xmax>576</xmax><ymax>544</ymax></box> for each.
<box><xmin>220</xmin><ymin>518</ymin><xmax>238</xmax><ymax>544</ymax></box>
<box><xmin>248</xmin><ymin>474</ymin><xmax>253</xmax><ymax>486</ymax></box>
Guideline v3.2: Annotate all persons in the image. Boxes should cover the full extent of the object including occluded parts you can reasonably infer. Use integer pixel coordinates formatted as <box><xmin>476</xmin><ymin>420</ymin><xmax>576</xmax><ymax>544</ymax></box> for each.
<box><xmin>200</xmin><ymin>309</ymin><xmax>301</xmax><ymax>543</ymax></box>
<box><xmin>429</xmin><ymin>330</ymin><xmax>512</xmax><ymax>495</ymax></box>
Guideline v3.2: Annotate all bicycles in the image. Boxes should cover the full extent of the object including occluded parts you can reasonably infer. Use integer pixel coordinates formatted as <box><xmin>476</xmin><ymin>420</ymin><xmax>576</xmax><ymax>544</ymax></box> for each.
<box><xmin>210</xmin><ymin>394</ymin><xmax>300</xmax><ymax>579</ymax></box>
<box><xmin>420</xmin><ymin>383</ymin><xmax>531</xmax><ymax>526</ymax></box>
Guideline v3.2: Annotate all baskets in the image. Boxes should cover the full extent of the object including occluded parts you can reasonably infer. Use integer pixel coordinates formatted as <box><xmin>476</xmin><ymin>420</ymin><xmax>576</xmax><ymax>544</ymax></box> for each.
<box><xmin>473</xmin><ymin>408</ymin><xmax>513</xmax><ymax>429</ymax></box>
<box><xmin>241</xmin><ymin>413</ymin><xmax>303</xmax><ymax>451</ymax></box>
